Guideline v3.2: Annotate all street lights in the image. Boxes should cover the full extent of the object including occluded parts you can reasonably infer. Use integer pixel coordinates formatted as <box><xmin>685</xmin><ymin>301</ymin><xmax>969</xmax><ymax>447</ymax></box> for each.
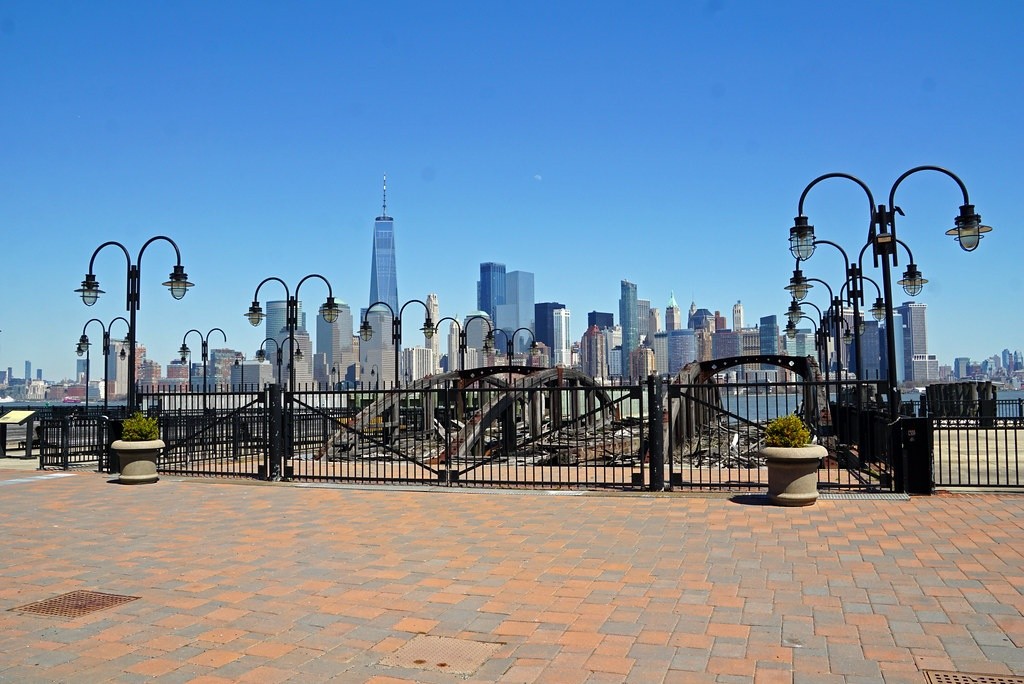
<box><xmin>356</xmin><ymin>299</ymin><xmax>439</xmax><ymax>389</ymax></box>
<box><xmin>74</xmin><ymin>315</ymin><xmax>132</xmax><ymax>413</ymax></box>
<box><xmin>786</xmin><ymin>166</ymin><xmax>994</xmax><ymax>417</ymax></box>
<box><xmin>255</xmin><ymin>336</ymin><xmax>304</xmax><ymax>385</ymax></box>
<box><xmin>72</xmin><ymin>235</ymin><xmax>195</xmax><ymax>416</ymax></box>
<box><xmin>781</xmin><ymin>237</ymin><xmax>932</xmax><ymax>415</ymax></box>
<box><xmin>431</xmin><ymin>315</ymin><xmax>493</xmax><ymax>368</ymax></box>
<box><xmin>243</xmin><ymin>274</ymin><xmax>342</xmax><ymax>482</ymax></box>
<box><xmin>176</xmin><ymin>328</ymin><xmax>227</xmax><ymax>414</ymax></box>
<box><xmin>481</xmin><ymin>327</ymin><xmax>540</xmax><ymax>386</ymax></box>
